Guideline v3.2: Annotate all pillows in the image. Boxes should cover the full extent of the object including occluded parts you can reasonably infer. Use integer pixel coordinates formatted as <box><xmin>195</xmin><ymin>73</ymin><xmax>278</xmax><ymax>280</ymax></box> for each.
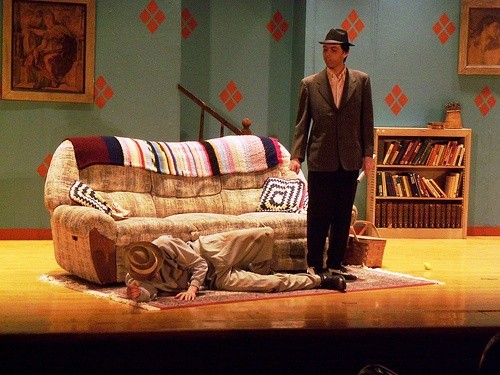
<box><xmin>257</xmin><ymin>177</ymin><xmax>306</xmax><ymax>213</ymax></box>
<box><xmin>70</xmin><ymin>177</ymin><xmax>112</xmax><ymax>217</ymax></box>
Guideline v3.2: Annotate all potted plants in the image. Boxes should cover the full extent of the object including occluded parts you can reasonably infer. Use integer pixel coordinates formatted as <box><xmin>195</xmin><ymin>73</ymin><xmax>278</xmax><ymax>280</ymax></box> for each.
<box><xmin>445</xmin><ymin>101</ymin><xmax>464</xmax><ymax>129</ymax></box>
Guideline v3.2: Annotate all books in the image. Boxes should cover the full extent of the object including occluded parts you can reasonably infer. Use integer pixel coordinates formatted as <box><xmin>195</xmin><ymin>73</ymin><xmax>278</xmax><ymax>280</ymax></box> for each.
<box><xmin>375</xmin><ymin>138</ymin><xmax>465</xmax><ymax>231</ymax></box>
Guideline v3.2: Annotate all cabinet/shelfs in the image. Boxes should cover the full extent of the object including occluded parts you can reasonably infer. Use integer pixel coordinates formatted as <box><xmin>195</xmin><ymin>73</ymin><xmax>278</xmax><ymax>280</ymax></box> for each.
<box><xmin>366</xmin><ymin>126</ymin><xmax>473</xmax><ymax>239</ymax></box>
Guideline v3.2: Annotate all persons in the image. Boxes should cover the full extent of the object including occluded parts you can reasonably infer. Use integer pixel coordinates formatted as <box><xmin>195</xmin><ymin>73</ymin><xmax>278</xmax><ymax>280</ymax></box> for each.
<box><xmin>289</xmin><ymin>27</ymin><xmax>375</xmax><ymax>278</ymax></box>
<box><xmin>125</xmin><ymin>227</ymin><xmax>347</xmax><ymax>303</ymax></box>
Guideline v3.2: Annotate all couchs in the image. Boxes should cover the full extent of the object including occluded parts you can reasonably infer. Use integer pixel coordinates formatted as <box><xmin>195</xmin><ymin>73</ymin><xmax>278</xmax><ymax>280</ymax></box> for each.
<box><xmin>44</xmin><ymin>134</ymin><xmax>308</xmax><ymax>285</ymax></box>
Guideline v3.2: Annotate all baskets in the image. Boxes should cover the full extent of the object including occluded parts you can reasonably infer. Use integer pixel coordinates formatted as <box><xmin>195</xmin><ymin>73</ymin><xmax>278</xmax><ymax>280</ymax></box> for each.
<box><xmin>343</xmin><ymin>219</ymin><xmax>386</xmax><ymax>269</ymax></box>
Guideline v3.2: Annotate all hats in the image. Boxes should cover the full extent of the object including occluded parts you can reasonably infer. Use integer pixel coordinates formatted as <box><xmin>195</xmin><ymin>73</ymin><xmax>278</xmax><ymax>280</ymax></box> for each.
<box><xmin>123</xmin><ymin>241</ymin><xmax>164</xmax><ymax>281</ymax></box>
<box><xmin>319</xmin><ymin>27</ymin><xmax>354</xmax><ymax>46</ymax></box>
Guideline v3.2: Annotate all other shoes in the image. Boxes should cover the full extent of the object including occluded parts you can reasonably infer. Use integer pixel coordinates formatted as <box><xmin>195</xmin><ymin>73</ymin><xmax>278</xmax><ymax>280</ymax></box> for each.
<box><xmin>307</xmin><ymin>266</ymin><xmax>324</xmax><ymax>277</ymax></box>
<box><xmin>328</xmin><ymin>265</ymin><xmax>358</xmax><ymax>281</ymax></box>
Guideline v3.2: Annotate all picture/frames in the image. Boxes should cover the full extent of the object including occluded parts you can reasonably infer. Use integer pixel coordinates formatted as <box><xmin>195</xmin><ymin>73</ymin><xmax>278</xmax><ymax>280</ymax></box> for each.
<box><xmin>458</xmin><ymin>0</ymin><xmax>500</xmax><ymax>75</ymax></box>
<box><xmin>1</xmin><ymin>0</ymin><xmax>96</xmax><ymax>104</ymax></box>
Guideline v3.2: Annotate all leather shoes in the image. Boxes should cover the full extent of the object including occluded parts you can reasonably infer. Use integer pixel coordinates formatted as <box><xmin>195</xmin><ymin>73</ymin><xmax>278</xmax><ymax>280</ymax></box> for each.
<box><xmin>319</xmin><ymin>271</ymin><xmax>346</xmax><ymax>290</ymax></box>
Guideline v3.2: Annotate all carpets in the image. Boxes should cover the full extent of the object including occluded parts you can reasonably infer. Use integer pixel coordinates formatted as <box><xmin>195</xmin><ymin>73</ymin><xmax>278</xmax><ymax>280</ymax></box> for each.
<box><xmin>45</xmin><ymin>263</ymin><xmax>437</xmax><ymax>311</ymax></box>
